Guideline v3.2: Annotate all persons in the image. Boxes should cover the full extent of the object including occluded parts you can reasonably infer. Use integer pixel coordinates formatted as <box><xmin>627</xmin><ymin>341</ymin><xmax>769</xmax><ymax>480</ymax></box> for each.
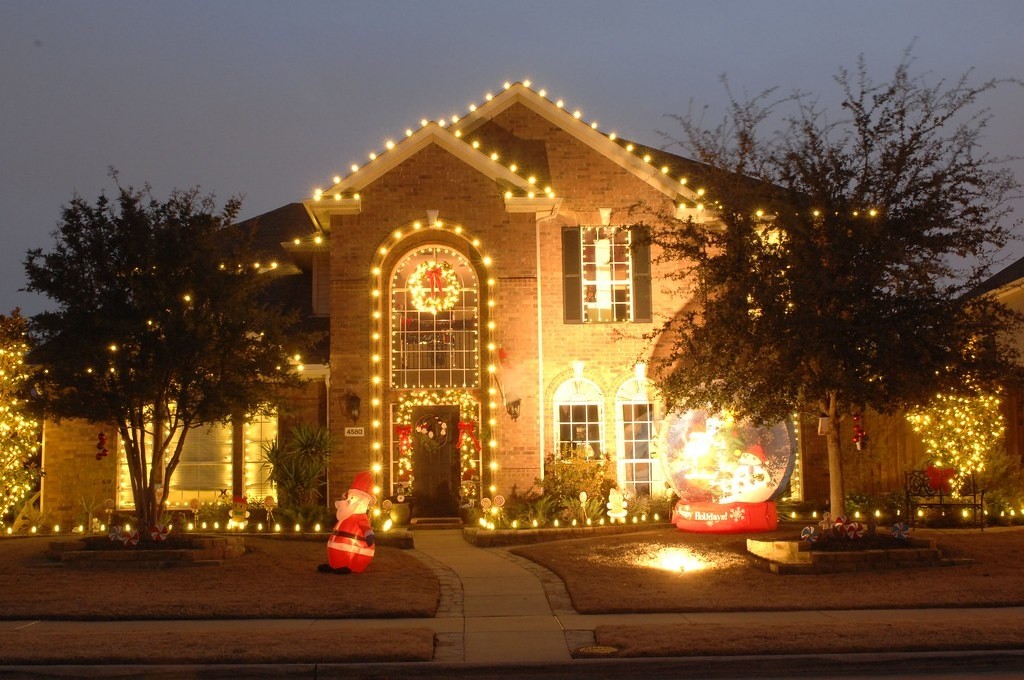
<box><xmin>327</xmin><ymin>473</ymin><xmax>376</xmax><ymax>573</ymax></box>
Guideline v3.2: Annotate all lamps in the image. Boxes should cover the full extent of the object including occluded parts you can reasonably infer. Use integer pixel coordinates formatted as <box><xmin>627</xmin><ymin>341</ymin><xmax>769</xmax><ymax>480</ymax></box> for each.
<box><xmin>348</xmin><ymin>391</ymin><xmax>360</xmax><ymax>424</ymax></box>
<box><xmin>509</xmin><ymin>399</ymin><xmax>520</xmax><ymax>422</ymax></box>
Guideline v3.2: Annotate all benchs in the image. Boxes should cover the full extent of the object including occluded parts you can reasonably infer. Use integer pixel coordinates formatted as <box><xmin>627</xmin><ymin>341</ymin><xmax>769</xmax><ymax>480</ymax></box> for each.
<box><xmin>904</xmin><ymin>466</ymin><xmax>989</xmax><ymax>532</ymax></box>
<box><xmin>105</xmin><ymin>508</ymin><xmax>198</xmax><ymax>532</ymax></box>
